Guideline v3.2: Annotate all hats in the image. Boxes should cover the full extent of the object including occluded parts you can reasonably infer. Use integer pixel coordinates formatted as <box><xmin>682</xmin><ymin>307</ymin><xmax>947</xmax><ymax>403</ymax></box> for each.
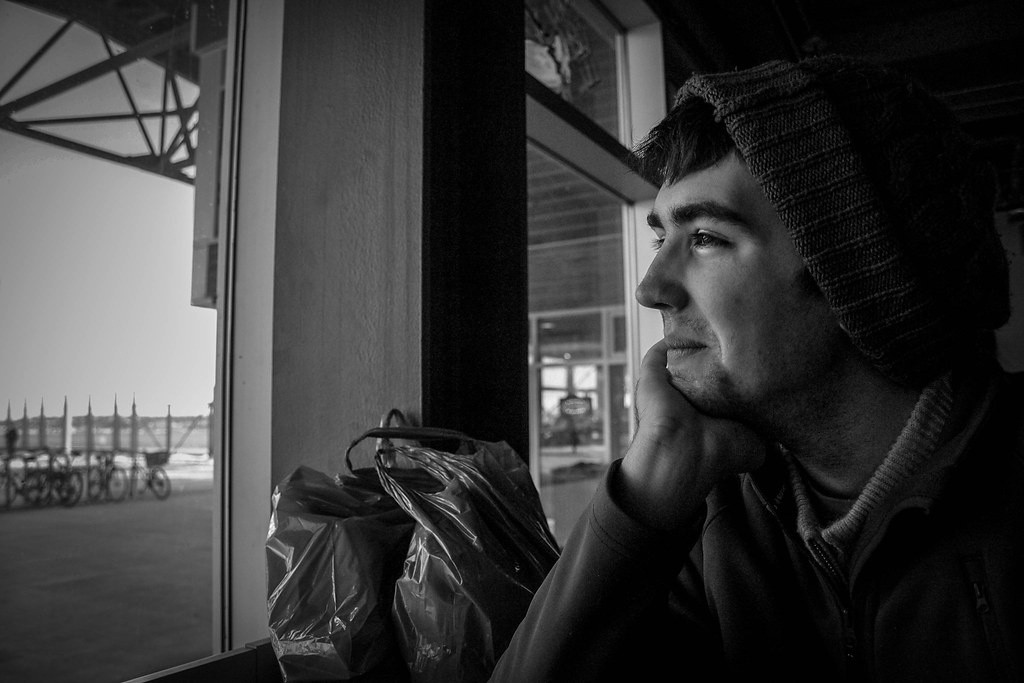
<box><xmin>670</xmin><ymin>51</ymin><xmax>1011</xmax><ymax>387</ymax></box>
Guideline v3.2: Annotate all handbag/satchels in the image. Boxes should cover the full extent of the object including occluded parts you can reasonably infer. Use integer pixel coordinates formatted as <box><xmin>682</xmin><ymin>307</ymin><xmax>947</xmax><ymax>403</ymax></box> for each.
<box><xmin>267</xmin><ymin>409</ymin><xmax>423</xmax><ymax>683</ymax></box>
<box><xmin>374</xmin><ymin>434</ymin><xmax>562</xmax><ymax>683</ymax></box>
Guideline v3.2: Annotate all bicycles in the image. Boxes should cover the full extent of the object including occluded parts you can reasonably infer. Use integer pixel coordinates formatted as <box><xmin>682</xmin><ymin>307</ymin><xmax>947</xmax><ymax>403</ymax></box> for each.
<box><xmin>0</xmin><ymin>449</ymin><xmax>171</xmax><ymax>510</ymax></box>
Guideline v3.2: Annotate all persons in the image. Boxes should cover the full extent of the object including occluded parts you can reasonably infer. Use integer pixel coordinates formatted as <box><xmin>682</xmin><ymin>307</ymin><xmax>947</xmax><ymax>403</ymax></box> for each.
<box><xmin>5</xmin><ymin>423</ymin><xmax>20</xmax><ymax>455</ymax></box>
<box><xmin>487</xmin><ymin>56</ymin><xmax>1024</xmax><ymax>683</ymax></box>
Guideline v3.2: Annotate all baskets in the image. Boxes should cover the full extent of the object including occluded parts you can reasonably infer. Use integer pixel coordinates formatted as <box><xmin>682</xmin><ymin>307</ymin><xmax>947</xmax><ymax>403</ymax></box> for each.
<box><xmin>145</xmin><ymin>452</ymin><xmax>169</xmax><ymax>467</ymax></box>
